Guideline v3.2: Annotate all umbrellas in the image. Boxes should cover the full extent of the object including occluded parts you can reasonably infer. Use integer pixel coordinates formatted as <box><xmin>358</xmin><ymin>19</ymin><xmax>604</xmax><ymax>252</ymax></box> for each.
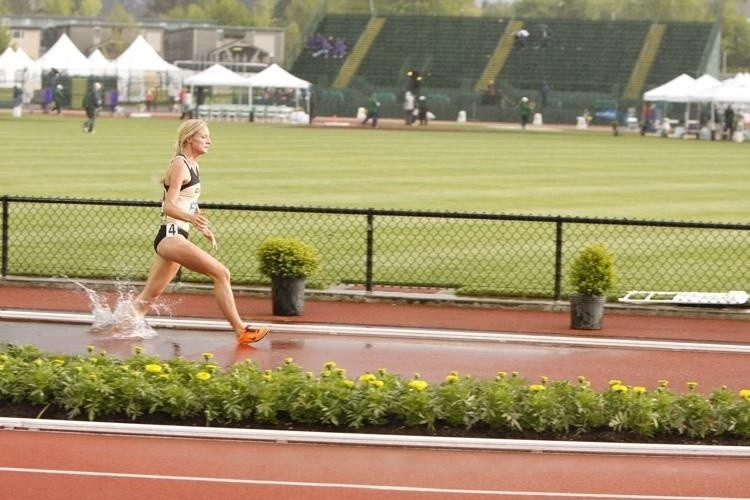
<box><xmin>0</xmin><ymin>35</ymin><xmax>311</xmax><ymax>132</ymax></box>
<box><xmin>641</xmin><ymin>70</ymin><xmax>750</xmax><ymax>121</ymax></box>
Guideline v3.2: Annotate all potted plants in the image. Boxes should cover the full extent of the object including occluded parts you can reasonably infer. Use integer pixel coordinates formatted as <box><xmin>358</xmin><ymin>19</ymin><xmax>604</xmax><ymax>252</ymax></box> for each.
<box><xmin>567</xmin><ymin>241</ymin><xmax>615</xmax><ymax>330</ymax></box>
<box><xmin>256</xmin><ymin>236</ymin><xmax>321</xmax><ymax>315</ymax></box>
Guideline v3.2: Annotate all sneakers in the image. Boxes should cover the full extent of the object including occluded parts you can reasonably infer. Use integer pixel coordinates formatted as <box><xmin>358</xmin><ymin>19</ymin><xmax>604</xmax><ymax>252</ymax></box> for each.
<box><xmin>237</xmin><ymin>326</ymin><xmax>271</xmax><ymax>346</ymax></box>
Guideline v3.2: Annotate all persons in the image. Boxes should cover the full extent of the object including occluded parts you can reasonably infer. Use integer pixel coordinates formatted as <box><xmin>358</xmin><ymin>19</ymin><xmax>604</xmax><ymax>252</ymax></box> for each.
<box><xmin>522</xmin><ymin>97</ymin><xmax>531</xmax><ymax>127</ymax></box>
<box><xmin>640</xmin><ymin>102</ymin><xmax>670</xmax><ymax>138</ymax></box>
<box><xmin>539</xmin><ymin>81</ymin><xmax>552</xmax><ymax>108</ymax></box>
<box><xmin>132</xmin><ymin>119</ymin><xmax>270</xmax><ymax>346</ymax></box>
<box><xmin>356</xmin><ymin>71</ymin><xmax>428</xmax><ymax>127</ymax></box>
<box><xmin>696</xmin><ymin>100</ymin><xmax>735</xmax><ymax>140</ymax></box>
<box><xmin>307</xmin><ymin>32</ymin><xmax>348</xmax><ymax>60</ymax></box>
<box><xmin>13</xmin><ymin>80</ymin><xmax>206</xmax><ymax>133</ymax></box>
